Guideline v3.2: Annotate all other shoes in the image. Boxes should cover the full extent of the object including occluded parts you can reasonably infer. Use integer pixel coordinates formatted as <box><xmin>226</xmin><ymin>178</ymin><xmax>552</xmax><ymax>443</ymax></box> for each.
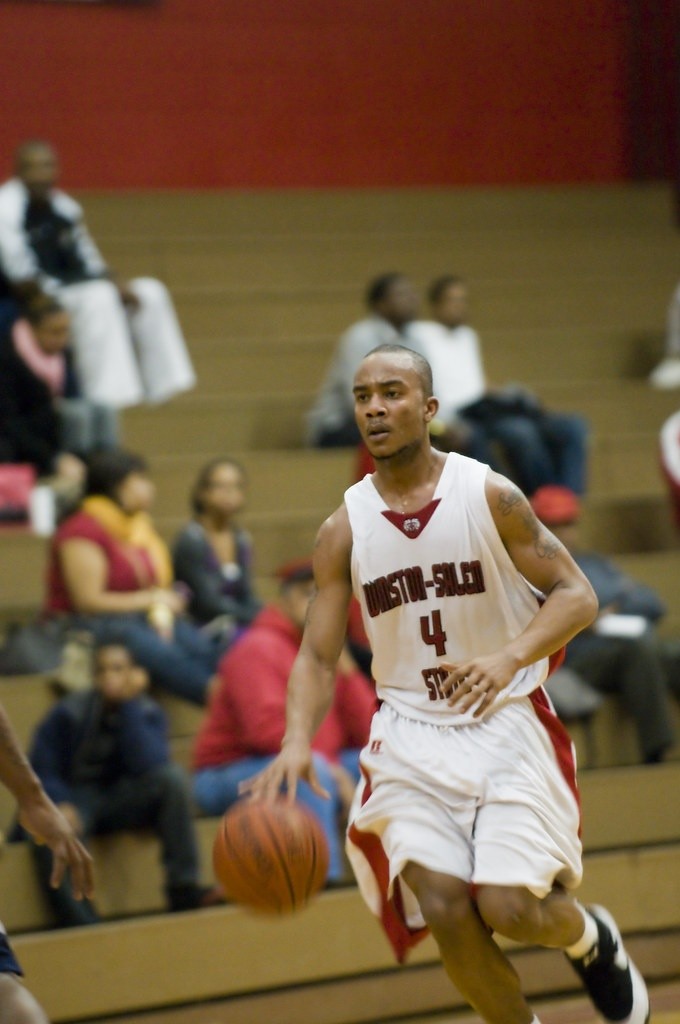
<box><xmin>168</xmin><ymin>883</ymin><xmax>222</xmax><ymax>911</ymax></box>
<box><xmin>50</xmin><ymin>909</ymin><xmax>95</xmax><ymax>927</ymax></box>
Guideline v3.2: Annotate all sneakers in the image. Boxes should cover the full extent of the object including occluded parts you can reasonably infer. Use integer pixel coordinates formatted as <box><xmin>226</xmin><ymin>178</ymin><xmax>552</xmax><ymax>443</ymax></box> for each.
<box><xmin>564</xmin><ymin>904</ymin><xmax>650</xmax><ymax>1024</ymax></box>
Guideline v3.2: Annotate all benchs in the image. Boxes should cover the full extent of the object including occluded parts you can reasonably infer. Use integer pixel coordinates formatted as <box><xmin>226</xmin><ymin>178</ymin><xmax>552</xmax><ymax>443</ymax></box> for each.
<box><xmin>0</xmin><ymin>188</ymin><xmax>680</xmax><ymax>1024</ymax></box>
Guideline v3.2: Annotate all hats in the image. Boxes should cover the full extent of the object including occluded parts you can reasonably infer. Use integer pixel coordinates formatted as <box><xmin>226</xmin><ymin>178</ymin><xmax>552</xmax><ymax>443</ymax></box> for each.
<box><xmin>531</xmin><ymin>485</ymin><xmax>577</xmax><ymax>522</ymax></box>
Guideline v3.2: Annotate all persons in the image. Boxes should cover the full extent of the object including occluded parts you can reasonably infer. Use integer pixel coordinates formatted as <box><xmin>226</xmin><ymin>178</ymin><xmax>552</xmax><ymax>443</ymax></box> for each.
<box><xmin>531</xmin><ymin>485</ymin><xmax>680</xmax><ymax>767</ymax></box>
<box><xmin>0</xmin><ymin>706</ymin><xmax>97</xmax><ymax>1024</ymax></box>
<box><xmin>189</xmin><ymin>565</ymin><xmax>379</xmax><ymax>889</ymax></box>
<box><xmin>236</xmin><ymin>345</ymin><xmax>652</xmax><ymax>1024</ymax></box>
<box><xmin>0</xmin><ymin>140</ymin><xmax>196</xmax><ymax>525</ymax></box>
<box><xmin>43</xmin><ymin>451</ymin><xmax>227</xmax><ymax>709</ymax></box>
<box><xmin>6</xmin><ymin>640</ymin><xmax>227</xmax><ymax>927</ymax></box>
<box><xmin>171</xmin><ymin>458</ymin><xmax>261</xmax><ymax>627</ymax></box>
<box><xmin>300</xmin><ymin>271</ymin><xmax>592</xmax><ymax>528</ymax></box>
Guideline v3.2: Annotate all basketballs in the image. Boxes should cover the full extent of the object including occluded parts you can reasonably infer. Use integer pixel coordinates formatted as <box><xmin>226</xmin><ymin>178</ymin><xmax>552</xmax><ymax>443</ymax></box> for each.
<box><xmin>211</xmin><ymin>792</ymin><xmax>328</xmax><ymax>915</ymax></box>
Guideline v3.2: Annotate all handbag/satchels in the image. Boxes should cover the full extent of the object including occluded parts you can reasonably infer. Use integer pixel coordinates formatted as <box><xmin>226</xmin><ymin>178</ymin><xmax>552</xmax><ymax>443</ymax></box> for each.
<box><xmin>0</xmin><ymin>612</ymin><xmax>61</xmax><ymax>674</ymax></box>
<box><xmin>0</xmin><ymin>462</ymin><xmax>34</xmax><ymax>528</ymax></box>
